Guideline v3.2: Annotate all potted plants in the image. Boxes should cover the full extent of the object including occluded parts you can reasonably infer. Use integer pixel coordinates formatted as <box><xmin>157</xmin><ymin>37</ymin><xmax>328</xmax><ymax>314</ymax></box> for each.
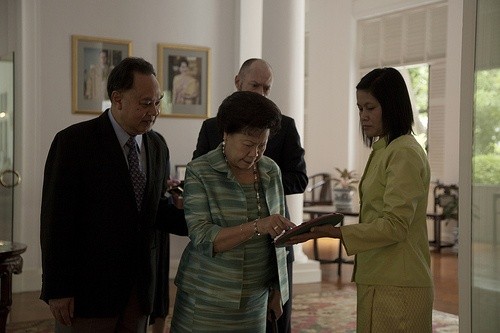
<box><xmin>325</xmin><ymin>168</ymin><xmax>360</xmax><ymax>211</ymax></box>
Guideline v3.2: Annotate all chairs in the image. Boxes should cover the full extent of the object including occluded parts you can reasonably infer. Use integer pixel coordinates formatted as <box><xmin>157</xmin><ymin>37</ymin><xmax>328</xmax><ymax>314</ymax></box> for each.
<box><xmin>304</xmin><ymin>173</ymin><xmax>331</xmax><ymax>206</ymax></box>
<box><xmin>425</xmin><ymin>183</ymin><xmax>459</xmax><ymax>248</ymax></box>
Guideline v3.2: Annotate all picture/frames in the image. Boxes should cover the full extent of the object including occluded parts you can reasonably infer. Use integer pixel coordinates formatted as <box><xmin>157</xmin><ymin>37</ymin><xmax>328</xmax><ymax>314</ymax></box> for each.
<box><xmin>71</xmin><ymin>34</ymin><xmax>132</xmax><ymax>115</ymax></box>
<box><xmin>156</xmin><ymin>43</ymin><xmax>210</xmax><ymax>118</ymax></box>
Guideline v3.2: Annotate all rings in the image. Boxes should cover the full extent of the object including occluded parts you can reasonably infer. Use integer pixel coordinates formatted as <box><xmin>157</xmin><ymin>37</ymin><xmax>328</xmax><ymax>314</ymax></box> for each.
<box><xmin>274</xmin><ymin>225</ymin><xmax>279</xmax><ymax>230</ymax></box>
<box><xmin>288</xmin><ymin>226</ymin><xmax>292</xmax><ymax>230</ymax></box>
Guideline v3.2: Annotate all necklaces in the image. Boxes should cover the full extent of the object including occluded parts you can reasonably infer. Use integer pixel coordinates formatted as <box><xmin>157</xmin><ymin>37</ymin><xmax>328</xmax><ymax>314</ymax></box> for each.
<box><xmin>223</xmin><ymin>144</ymin><xmax>261</xmax><ymax>219</ymax></box>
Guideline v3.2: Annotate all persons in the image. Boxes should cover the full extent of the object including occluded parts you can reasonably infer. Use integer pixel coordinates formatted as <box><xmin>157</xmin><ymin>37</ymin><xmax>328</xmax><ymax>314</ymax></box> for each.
<box><xmin>87</xmin><ymin>50</ymin><xmax>115</xmax><ymax>100</ymax></box>
<box><xmin>284</xmin><ymin>67</ymin><xmax>433</xmax><ymax>333</ymax></box>
<box><xmin>168</xmin><ymin>90</ymin><xmax>296</xmax><ymax>333</ymax></box>
<box><xmin>172</xmin><ymin>57</ymin><xmax>199</xmax><ymax>106</ymax></box>
<box><xmin>192</xmin><ymin>59</ymin><xmax>308</xmax><ymax>332</ymax></box>
<box><xmin>41</xmin><ymin>56</ymin><xmax>188</xmax><ymax>333</ymax></box>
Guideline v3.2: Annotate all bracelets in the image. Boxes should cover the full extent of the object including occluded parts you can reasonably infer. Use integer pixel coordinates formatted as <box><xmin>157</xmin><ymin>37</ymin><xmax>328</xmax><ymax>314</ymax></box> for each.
<box><xmin>253</xmin><ymin>219</ymin><xmax>260</xmax><ymax>236</ymax></box>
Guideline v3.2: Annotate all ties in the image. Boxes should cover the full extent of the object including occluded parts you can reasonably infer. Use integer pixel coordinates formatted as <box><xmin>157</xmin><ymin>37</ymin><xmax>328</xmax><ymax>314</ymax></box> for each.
<box><xmin>126</xmin><ymin>135</ymin><xmax>149</xmax><ymax>215</ymax></box>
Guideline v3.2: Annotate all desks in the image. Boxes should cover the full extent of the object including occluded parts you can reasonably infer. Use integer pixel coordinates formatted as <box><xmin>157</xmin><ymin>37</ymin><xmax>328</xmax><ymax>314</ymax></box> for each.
<box><xmin>303</xmin><ymin>207</ymin><xmax>361</xmax><ymax>284</ymax></box>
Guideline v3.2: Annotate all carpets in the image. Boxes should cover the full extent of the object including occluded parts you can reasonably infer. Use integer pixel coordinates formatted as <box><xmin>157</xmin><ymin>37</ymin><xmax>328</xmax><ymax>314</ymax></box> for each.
<box><xmin>6</xmin><ymin>288</ymin><xmax>459</xmax><ymax>333</ymax></box>
